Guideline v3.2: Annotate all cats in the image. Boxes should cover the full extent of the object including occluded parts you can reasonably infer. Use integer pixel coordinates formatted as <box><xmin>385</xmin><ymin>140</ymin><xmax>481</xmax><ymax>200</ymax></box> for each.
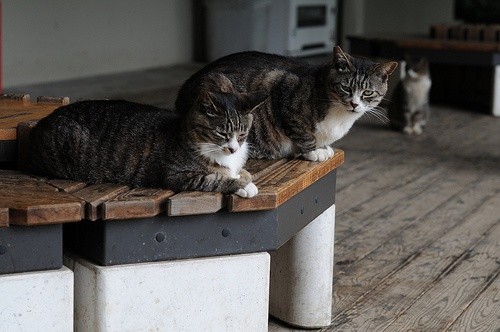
<box><xmin>26</xmin><ymin>88</ymin><xmax>267</xmax><ymax>195</ymax></box>
<box><xmin>388</xmin><ymin>57</ymin><xmax>431</xmax><ymax>130</ymax></box>
<box><xmin>176</xmin><ymin>45</ymin><xmax>397</xmax><ymax>162</ymax></box>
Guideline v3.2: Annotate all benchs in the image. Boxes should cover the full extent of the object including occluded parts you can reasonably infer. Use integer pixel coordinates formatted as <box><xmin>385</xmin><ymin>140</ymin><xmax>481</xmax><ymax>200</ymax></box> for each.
<box><xmin>346</xmin><ymin>33</ymin><xmax>500</xmax><ymax>118</ymax></box>
<box><xmin>0</xmin><ymin>97</ymin><xmax>344</xmax><ymax>332</ymax></box>
